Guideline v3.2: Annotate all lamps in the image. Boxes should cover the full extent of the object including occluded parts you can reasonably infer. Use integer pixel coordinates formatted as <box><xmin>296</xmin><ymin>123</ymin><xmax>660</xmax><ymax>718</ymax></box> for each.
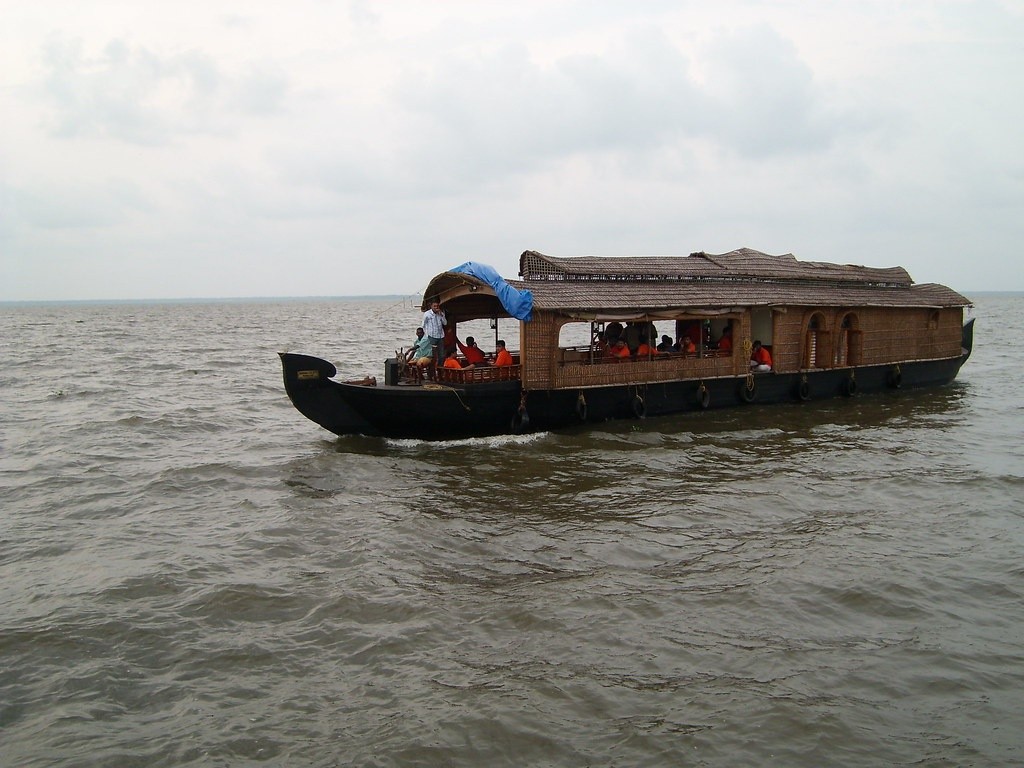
<box><xmin>490</xmin><ymin>312</ymin><xmax>497</xmax><ymax>330</ymax></box>
<box><xmin>592</xmin><ymin>320</ymin><xmax>600</xmax><ymax>334</ymax></box>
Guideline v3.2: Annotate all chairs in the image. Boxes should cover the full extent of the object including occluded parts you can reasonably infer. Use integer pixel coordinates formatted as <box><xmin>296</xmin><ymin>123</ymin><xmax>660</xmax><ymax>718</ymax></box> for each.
<box><xmin>410</xmin><ymin>352</ymin><xmax>440</xmax><ymax>385</ymax></box>
<box><xmin>556</xmin><ymin>348</ymin><xmax>732</xmax><ymax>365</ymax></box>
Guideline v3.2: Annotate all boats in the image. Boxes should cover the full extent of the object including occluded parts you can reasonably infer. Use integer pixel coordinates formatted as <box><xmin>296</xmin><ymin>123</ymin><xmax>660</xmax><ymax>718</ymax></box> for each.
<box><xmin>273</xmin><ymin>247</ymin><xmax>980</xmax><ymax>443</ymax></box>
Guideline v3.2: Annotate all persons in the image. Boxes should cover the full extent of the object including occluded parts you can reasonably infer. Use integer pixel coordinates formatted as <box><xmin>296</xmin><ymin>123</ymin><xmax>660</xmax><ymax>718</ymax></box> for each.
<box><xmin>595</xmin><ymin>321</ymin><xmax>670</xmax><ymax>358</ymax></box>
<box><xmin>750</xmin><ymin>340</ymin><xmax>772</xmax><ymax>372</ymax></box>
<box><xmin>421</xmin><ymin>299</ymin><xmax>447</xmax><ymax>378</ymax></box>
<box><xmin>473</xmin><ymin>342</ymin><xmax>485</xmax><ymax>357</ymax></box>
<box><xmin>443</xmin><ymin>346</ymin><xmax>475</xmax><ymax>380</ymax></box>
<box><xmin>456</xmin><ymin>336</ymin><xmax>486</xmax><ymax>367</ymax></box>
<box><xmin>491</xmin><ymin>340</ymin><xmax>513</xmax><ymax>367</ymax></box>
<box><xmin>657</xmin><ymin>335</ymin><xmax>695</xmax><ymax>353</ymax></box>
<box><xmin>405</xmin><ymin>328</ymin><xmax>432</xmax><ymax>383</ymax></box>
<box><xmin>717</xmin><ymin>326</ymin><xmax>732</xmax><ymax>357</ymax></box>
